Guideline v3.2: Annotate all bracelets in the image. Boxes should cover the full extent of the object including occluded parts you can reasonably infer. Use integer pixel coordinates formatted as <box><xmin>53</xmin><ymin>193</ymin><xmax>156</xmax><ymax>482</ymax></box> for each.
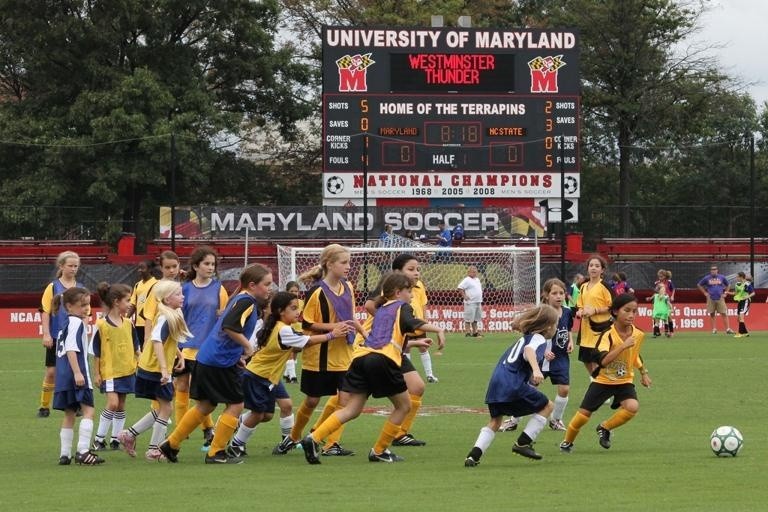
<box><xmin>639</xmin><ymin>367</ymin><xmax>648</xmax><ymax>376</ymax></box>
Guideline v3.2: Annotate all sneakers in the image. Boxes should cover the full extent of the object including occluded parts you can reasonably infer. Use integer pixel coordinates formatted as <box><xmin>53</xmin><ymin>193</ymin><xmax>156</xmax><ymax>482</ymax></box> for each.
<box><xmin>392</xmin><ymin>434</ymin><xmax>426</xmax><ymax>446</ymax></box>
<box><xmin>595</xmin><ymin>423</ymin><xmax>612</xmax><ymax>449</ymax></box>
<box><xmin>271</xmin><ymin>433</ymin><xmax>355</xmax><ymax>464</ymax></box>
<box><xmin>368</xmin><ymin>447</ymin><xmax>405</xmax><ymax>463</ymax></box>
<box><xmin>463</xmin><ymin>331</ymin><xmax>482</xmax><ymax>337</ymax></box>
<box><xmin>58</xmin><ymin>415</ymin><xmax>249</xmax><ymax>467</ymax></box>
<box><xmin>651</xmin><ymin>325</ymin><xmax>751</xmax><ymax>339</ymax></box>
<box><xmin>36</xmin><ymin>407</ymin><xmax>50</xmax><ymax>417</ymax></box>
<box><xmin>558</xmin><ymin>441</ymin><xmax>573</xmax><ymax>451</ymax></box>
<box><xmin>282</xmin><ymin>373</ymin><xmax>300</xmax><ymax>385</ymax></box>
<box><xmin>549</xmin><ymin>419</ymin><xmax>567</xmax><ymax>431</ymax></box>
<box><xmin>497</xmin><ymin>418</ymin><xmax>518</xmax><ymax>432</ymax></box>
<box><xmin>426</xmin><ymin>375</ymin><xmax>439</xmax><ymax>384</ymax></box>
<box><xmin>510</xmin><ymin>440</ymin><xmax>542</xmax><ymax>461</ymax></box>
<box><xmin>464</xmin><ymin>453</ymin><xmax>478</xmax><ymax>466</ymax></box>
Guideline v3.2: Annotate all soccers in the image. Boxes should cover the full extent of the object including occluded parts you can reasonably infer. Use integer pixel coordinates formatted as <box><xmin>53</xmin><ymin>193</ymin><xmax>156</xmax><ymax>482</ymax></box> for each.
<box><xmin>709</xmin><ymin>425</ymin><xmax>745</xmax><ymax>458</ymax></box>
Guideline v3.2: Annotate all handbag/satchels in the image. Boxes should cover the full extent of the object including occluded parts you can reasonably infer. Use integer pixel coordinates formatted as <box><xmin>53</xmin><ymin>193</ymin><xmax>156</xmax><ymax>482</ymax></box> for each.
<box><xmin>589</xmin><ymin>319</ymin><xmax>611</xmax><ymax>335</ymax></box>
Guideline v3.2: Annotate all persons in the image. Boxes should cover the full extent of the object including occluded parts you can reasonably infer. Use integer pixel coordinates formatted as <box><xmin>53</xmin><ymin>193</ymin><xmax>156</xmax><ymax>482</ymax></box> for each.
<box><xmin>560</xmin><ymin>292</ymin><xmax>651</xmax><ymax>452</ymax></box>
<box><xmin>496</xmin><ymin>276</ymin><xmax>574</xmax><ymax>432</ymax></box>
<box><xmin>565</xmin><ymin>264</ymin><xmax>677</xmax><ymax>341</ymax></box>
<box><xmin>722</xmin><ymin>271</ymin><xmax>756</xmax><ymax>338</ymax></box>
<box><xmin>455</xmin><ymin>264</ymin><xmax>484</xmax><ymax>338</ymax></box>
<box><xmin>463</xmin><ymin>302</ymin><xmax>560</xmax><ymax>468</ymax></box>
<box><xmin>435</xmin><ymin>223</ymin><xmax>452</xmax><ymax>247</ymax></box>
<box><xmin>380</xmin><ymin>224</ymin><xmax>400</xmax><ymax>248</ymax></box>
<box><xmin>404</xmin><ymin>229</ymin><xmax>415</xmax><ymax>242</ymax></box>
<box><xmin>695</xmin><ymin>263</ymin><xmax>735</xmax><ymax>335</ymax></box>
<box><xmin>576</xmin><ymin>255</ymin><xmax>615</xmax><ymax>380</ymax></box>
